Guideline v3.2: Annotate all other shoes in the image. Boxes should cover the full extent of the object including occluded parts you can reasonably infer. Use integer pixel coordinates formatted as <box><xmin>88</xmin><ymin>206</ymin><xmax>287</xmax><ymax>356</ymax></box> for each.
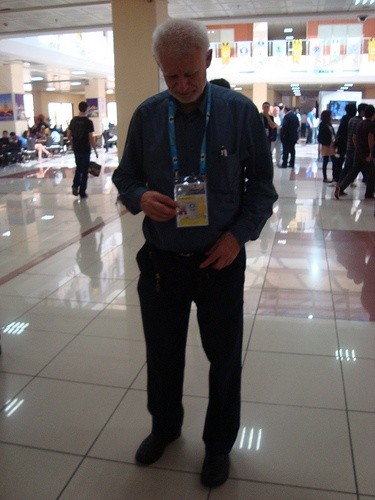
<box><xmin>80</xmin><ymin>194</ymin><xmax>88</xmax><ymax>198</ymax></box>
<box><xmin>72</xmin><ymin>186</ymin><xmax>78</xmax><ymax>196</ymax></box>
<box><xmin>328</xmin><ymin>181</ymin><xmax>339</xmax><ymax>187</ymax></box>
<box><xmin>365</xmin><ymin>192</ymin><xmax>375</xmax><ymax>198</ymax></box>
<box><xmin>340</xmin><ymin>191</ymin><xmax>347</xmax><ymax>195</ymax></box>
<box><xmin>335</xmin><ymin>186</ymin><xmax>340</xmax><ymax>199</ymax></box>
<box><xmin>135</xmin><ymin>428</ymin><xmax>181</xmax><ymax>465</ymax></box>
<box><xmin>200</xmin><ymin>445</ymin><xmax>229</xmax><ymax>487</ymax></box>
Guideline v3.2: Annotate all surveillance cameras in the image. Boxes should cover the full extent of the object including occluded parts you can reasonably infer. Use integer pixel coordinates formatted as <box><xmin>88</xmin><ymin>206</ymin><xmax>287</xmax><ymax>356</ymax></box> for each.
<box><xmin>359</xmin><ymin>17</ymin><xmax>365</xmax><ymax>21</ymax></box>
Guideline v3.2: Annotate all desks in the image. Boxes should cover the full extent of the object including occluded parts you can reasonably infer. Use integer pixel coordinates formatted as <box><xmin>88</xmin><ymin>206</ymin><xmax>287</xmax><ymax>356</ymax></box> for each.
<box><xmin>92</xmin><ymin>134</ymin><xmax>101</xmax><ymax>148</ymax></box>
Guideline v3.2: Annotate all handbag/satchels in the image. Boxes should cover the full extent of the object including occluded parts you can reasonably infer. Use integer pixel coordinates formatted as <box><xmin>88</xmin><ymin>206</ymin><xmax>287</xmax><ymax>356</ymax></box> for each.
<box><xmin>87</xmin><ymin>162</ymin><xmax>101</xmax><ymax>176</ymax></box>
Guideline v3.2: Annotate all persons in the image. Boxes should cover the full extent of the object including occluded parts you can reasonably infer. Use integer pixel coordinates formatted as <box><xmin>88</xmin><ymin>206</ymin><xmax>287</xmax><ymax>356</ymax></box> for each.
<box><xmin>66</xmin><ymin>101</ymin><xmax>98</xmax><ymax>198</ymax></box>
<box><xmin>295</xmin><ymin>108</ymin><xmax>301</xmax><ymax>144</ymax></box>
<box><xmin>317</xmin><ymin>109</ymin><xmax>336</xmax><ymax>183</ymax></box>
<box><xmin>278</xmin><ymin>105</ymin><xmax>299</xmax><ymax>168</ymax></box>
<box><xmin>259</xmin><ymin>102</ymin><xmax>276</xmax><ymax>147</ymax></box>
<box><xmin>306</xmin><ymin>106</ymin><xmax>316</xmax><ymax>144</ymax></box>
<box><xmin>0</xmin><ymin>130</ymin><xmax>10</xmax><ymax>166</ymax></box>
<box><xmin>22</xmin><ymin>114</ymin><xmax>53</xmax><ymax>161</ymax></box>
<box><xmin>271</xmin><ymin>103</ymin><xmax>285</xmax><ymax>164</ymax></box>
<box><xmin>51</xmin><ymin>124</ymin><xmax>63</xmax><ymax>133</ymax></box>
<box><xmin>9</xmin><ymin>132</ymin><xmax>27</xmax><ymax>152</ymax></box>
<box><xmin>210</xmin><ymin>78</ymin><xmax>231</xmax><ymax>89</ymax></box>
<box><xmin>301</xmin><ymin>111</ymin><xmax>306</xmax><ymax>138</ymax></box>
<box><xmin>336</xmin><ymin>104</ymin><xmax>357</xmax><ymax>188</ymax></box>
<box><xmin>339</xmin><ymin>103</ymin><xmax>368</xmax><ymax>196</ymax></box>
<box><xmin>334</xmin><ymin>104</ymin><xmax>375</xmax><ymax>200</ymax></box>
<box><xmin>111</xmin><ymin>15</ymin><xmax>278</xmax><ymax>486</ymax></box>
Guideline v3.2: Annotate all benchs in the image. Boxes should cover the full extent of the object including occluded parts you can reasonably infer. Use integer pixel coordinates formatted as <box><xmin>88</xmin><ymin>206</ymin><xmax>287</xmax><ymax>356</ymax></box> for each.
<box><xmin>24</xmin><ymin>145</ymin><xmax>64</xmax><ymax>161</ymax></box>
